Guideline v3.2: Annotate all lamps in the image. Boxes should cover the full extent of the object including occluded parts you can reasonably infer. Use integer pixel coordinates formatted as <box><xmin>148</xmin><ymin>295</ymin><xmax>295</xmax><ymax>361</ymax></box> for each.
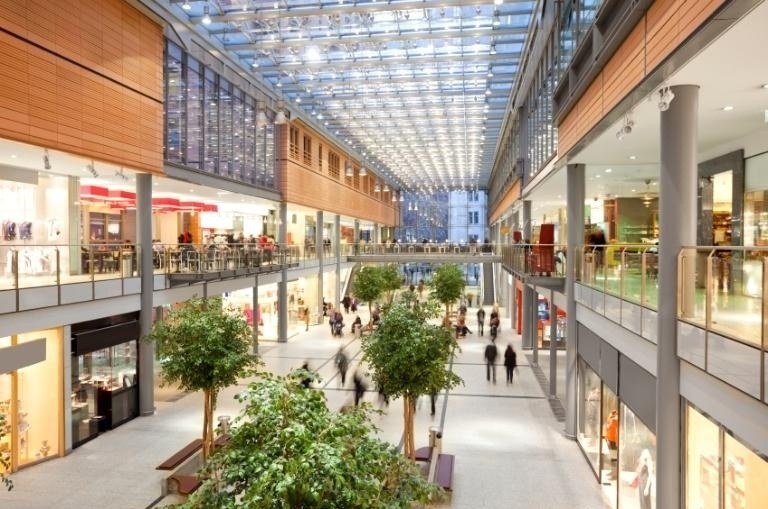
<box><xmin>223</xmin><ymin>28</ymin><xmax>229</xmax><ymax>41</ymax></box>
<box><xmin>116</xmin><ymin>168</ymin><xmax>128</xmax><ymax>180</ymax></box>
<box><xmin>659</xmin><ymin>83</ymin><xmax>674</xmax><ymax>110</ymax></box>
<box><xmin>479</xmin><ymin>82</ymin><xmax>492</xmax><ymax>154</ymax></box>
<box><xmin>359</xmin><ymin>155</ymin><xmax>367</xmax><ymax>175</ymax></box>
<box><xmin>42</xmin><ymin>149</ymin><xmax>50</xmax><ymax>169</ymax></box>
<box><xmin>276</xmin><ymin>76</ymin><xmax>316</xmax><ymax>115</ymax></box>
<box><xmin>346</xmin><ymin>154</ymin><xmax>353</xmax><ymax>177</ymax></box>
<box><xmin>373</xmin><ymin>174</ymin><xmax>379</xmax><ymax>191</ymax></box>
<box><xmin>182</xmin><ymin>0</ymin><xmax>191</xmax><ymax>9</ymax></box>
<box><xmin>201</xmin><ymin>6</ymin><xmax>211</xmax><ymax>24</ymax></box>
<box><xmin>487</xmin><ymin>65</ymin><xmax>493</xmax><ymax>76</ymax></box>
<box><xmin>252</xmin><ymin>53</ymin><xmax>259</xmax><ymax>67</ymax></box>
<box><xmin>392</xmin><ymin>191</ymin><xmax>396</xmax><ymax>201</ymax></box>
<box><xmin>399</xmin><ymin>191</ymin><xmax>404</xmax><ymax>201</ymax></box>
<box><xmin>616</xmin><ymin>110</ymin><xmax>636</xmax><ymax>139</ymax></box>
<box><xmin>492</xmin><ymin>11</ymin><xmax>500</xmax><ymax>26</ymax></box>
<box><xmin>87</xmin><ymin>160</ymin><xmax>97</xmax><ymax>176</ymax></box>
<box><xmin>382</xmin><ymin>180</ymin><xmax>389</xmax><ymax>191</ymax></box>
<box><xmin>490</xmin><ymin>41</ymin><xmax>497</xmax><ymax>54</ymax></box>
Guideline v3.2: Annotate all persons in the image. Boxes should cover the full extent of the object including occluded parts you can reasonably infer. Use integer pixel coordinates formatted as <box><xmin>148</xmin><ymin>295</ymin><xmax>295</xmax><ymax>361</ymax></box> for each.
<box><xmin>636</xmin><ymin>448</ymin><xmax>654</xmax><ymax>509</ymax></box>
<box><xmin>523</xmin><ymin>239</ymin><xmax>532</xmax><ymax>251</ymax></box>
<box><xmin>305</xmin><ymin>237</ymin><xmax>332</xmax><ymax>258</ymax></box>
<box><xmin>586</xmin><ymin>392</ymin><xmax>599</xmax><ymax>447</ymax></box>
<box><xmin>589</xmin><ymin>226</ymin><xmax>606</xmax><ymax>268</ymax></box>
<box><xmin>292</xmin><ymin>289</ymin><xmax>383</xmax><ymax>407</ymax></box>
<box><xmin>78</xmin><ymin>233</ymin><xmax>163</xmax><ymax>267</ymax></box>
<box><xmin>604</xmin><ymin>411</ymin><xmax>618</xmax><ymax>481</ymax></box>
<box><xmin>504</xmin><ymin>344</ymin><xmax>516</xmax><ymax>383</ymax></box>
<box><xmin>175</xmin><ymin>228</ymin><xmax>277</xmax><ymax>273</ymax></box>
<box><xmin>486</xmin><ymin>339</ymin><xmax>497</xmax><ymax>382</ymax></box>
<box><xmin>439</xmin><ymin>292</ymin><xmax>502</xmax><ymax>341</ymax></box>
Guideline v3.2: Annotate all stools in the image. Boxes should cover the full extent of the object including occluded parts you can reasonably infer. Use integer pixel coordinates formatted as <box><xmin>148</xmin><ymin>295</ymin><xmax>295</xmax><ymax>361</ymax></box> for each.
<box><xmin>394</xmin><ymin>246</ymin><xmax>460</xmax><ymax>254</ymax></box>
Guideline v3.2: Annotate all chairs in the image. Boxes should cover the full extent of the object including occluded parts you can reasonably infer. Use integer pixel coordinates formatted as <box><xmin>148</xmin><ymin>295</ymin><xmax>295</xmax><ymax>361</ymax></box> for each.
<box><xmin>82</xmin><ymin>247</ymin><xmax>239</xmax><ymax>274</ymax></box>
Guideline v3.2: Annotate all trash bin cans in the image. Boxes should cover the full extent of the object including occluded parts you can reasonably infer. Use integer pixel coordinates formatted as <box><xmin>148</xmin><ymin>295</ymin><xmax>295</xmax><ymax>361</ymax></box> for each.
<box><xmin>122</xmin><ymin>251</ymin><xmax>132</xmax><ymax>278</ymax></box>
<box><xmin>217</xmin><ymin>416</ymin><xmax>231</xmax><ymax>436</ymax></box>
<box><xmin>429</xmin><ymin>428</ymin><xmax>443</xmax><ymax>454</ymax></box>
<box><xmin>580</xmin><ymin>252</ymin><xmax>595</xmax><ymax>283</ymax></box>
<box><xmin>213</xmin><ymin>249</ymin><xmax>224</xmax><ymax>270</ymax></box>
<box><xmin>355</xmin><ymin>324</ymin><xmax>361</xmax><ymax>340</ymax></box>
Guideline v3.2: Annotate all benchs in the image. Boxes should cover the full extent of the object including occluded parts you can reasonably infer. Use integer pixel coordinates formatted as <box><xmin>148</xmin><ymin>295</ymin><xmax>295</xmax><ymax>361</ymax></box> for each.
<box><xmin>436</xmin><ymin>454</ymin><xmax>454</xmax><ymax>492</ymax></box>
<box><xmin>155</xmin><ymin>439</ymin><xmax>203</xmax><ymax>477</ymax></box>
